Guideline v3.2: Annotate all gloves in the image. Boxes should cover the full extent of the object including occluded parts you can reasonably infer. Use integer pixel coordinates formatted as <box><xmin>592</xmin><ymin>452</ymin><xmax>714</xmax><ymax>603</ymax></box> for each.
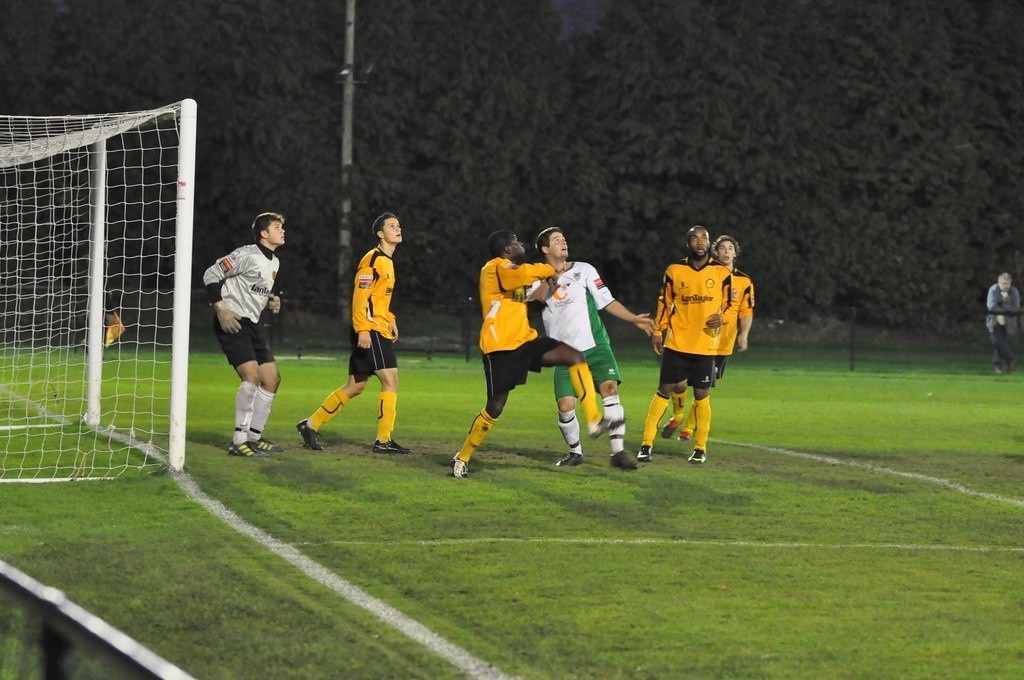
<box><xmin>215</xmin><ymin>302</ymin><xmax>243</xmax><ymax>334</ymax></box>
<box><xmin>267</xmin><ymin>295</ymin><xmax>281</xmax><ymax>315</ymax></box>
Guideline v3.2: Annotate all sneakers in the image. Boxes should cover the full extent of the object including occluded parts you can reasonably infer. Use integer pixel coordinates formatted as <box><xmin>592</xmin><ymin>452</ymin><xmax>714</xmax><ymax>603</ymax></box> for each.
<box><xmin>662</xmin><ymin>415</ymin><xmax>685</xmax><ymax>439</ymax></box>
<box><xmin>688</xmin><ymin>450</ymin><xmax>708</xmax><ymax>465</ymax></box>
<box><xmin>588</xmin><ymin>419</ymin><xmax>625</xmax><ymax>441</ymax></box>
<box><xmin>638</xmin><ymin>445</ymin><xmax>653</xmax><ymax>462</ymax></box>
<box><xmin>296</xmin><ymin>419</ymin><xmax>324</xmax><ymax>450</ymax></box>
<box><xmin>678</xmin><ymin>427</ymin><xmax>693</xmax><ymax>441</ymax></box>
<box><xmin>556</xmin><ymin>453</ymin><xmax>585</xmax><ymax>466</ymax></box>
<box><xmin>373</xmin><ymin>439</ymin><xmax>413</xmax><ymax>455</ymax></box>
<box><xmin>228</xmin><ymin>441</ymin><xmax>282</xmax><ymax>457</ymax></box>
<box><xmin>451</xmin><ymin>453</ymin><xmax>469</xmax><ymax>479</ymax></box>
<box><xmin>610</xmin><ymin>453</ymin><xmax>640</xmax><ymax>470</ymax></box>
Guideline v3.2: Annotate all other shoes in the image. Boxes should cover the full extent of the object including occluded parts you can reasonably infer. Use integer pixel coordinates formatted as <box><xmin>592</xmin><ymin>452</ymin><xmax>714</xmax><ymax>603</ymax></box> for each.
<box><xmin>993</xmin><ymin>359</ymin><xmax>1020</xmax><ymax>375</ymax></box>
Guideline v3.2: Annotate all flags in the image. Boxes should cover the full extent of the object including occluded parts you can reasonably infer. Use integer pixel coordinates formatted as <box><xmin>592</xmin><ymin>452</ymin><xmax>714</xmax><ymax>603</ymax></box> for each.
<box><xmin>105</xmin><ymin>320</ymin><xmax>125</xmax><ymax>349</ymax></box>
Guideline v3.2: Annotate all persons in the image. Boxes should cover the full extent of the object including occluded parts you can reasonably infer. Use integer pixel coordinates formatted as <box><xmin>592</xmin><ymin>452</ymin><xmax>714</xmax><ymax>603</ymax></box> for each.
<box><xmin>78</xmin><ymin>240</ymin><xmax>124</xmax><ymax>354</ymax></box>
<box><xmin>204</xmin><ymin>212</ymin><xmax>287</xmax><ymax>457</ymax></box>
<box><xmin>449</xmin><ymin>228</ymin><xmax>626</xmax><ymax>479</ymax></box>
<box><xmin>637</xmin><ymin>224</ymin><xmax>755</xmax><ymax>464</ymax></box>
<box><xmin>527</xmin><ymin>225</ymin><xmax>655</xmax><ymax>479</ymax></box>
<box><xmin>296</xmin><ymin>213</ymin><xmax>411</xmax><ymax>454</ymax></box>
<box><xmin>986</xmin><ymin>273</ymin><xmax>1020</xmax><ymax>376</ymax></box>
